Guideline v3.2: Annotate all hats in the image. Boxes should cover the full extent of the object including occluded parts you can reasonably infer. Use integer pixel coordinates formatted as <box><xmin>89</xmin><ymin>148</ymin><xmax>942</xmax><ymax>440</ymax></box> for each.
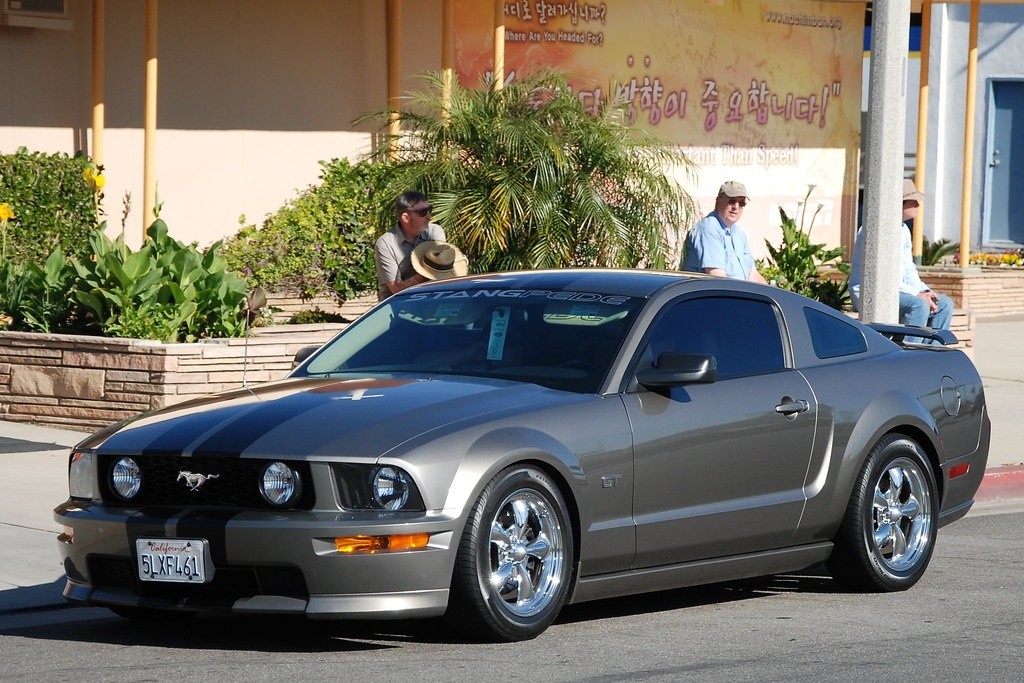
<box><xmin>718</xmin><ymin>180</ymin><xmax>752</xmax><ymax>202</ymax></box>
<box><xmin>903</xmin><ymin>178</ymin><xmax>926</xmax><ymax>200</ymax></box>
<box><xmin>410</xmin><ymin>241</ymin><xmax>469</xmax><ymax>281</ymax></box>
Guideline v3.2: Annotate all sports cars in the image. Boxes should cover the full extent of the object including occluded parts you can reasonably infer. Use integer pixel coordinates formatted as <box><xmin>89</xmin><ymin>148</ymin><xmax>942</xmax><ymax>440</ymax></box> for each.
<box><xmin>52</xmin><ymin>268</ymin><xmax>993</xmax><ymax>643</ymax></box>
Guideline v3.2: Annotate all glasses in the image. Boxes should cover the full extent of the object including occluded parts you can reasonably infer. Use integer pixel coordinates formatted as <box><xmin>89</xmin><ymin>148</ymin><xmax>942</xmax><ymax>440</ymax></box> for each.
<box><xmin>721</xmin><ymin>194</ymin><xmax>746</xmax><ymax>207</ymax></box>
<box><xmin>412</xmin><ymin>204</ymin><xmax>434</xmax><ymax>217</ymax></box>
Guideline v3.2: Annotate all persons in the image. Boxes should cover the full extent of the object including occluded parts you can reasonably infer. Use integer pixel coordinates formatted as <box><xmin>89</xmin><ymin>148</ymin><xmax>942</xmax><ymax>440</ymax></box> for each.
<box><xmin>372</xmin><ymin>190</ymin><xmax>447</xmax><ymax>303</ymax></box>
<box><xmin>678</xmin><ymin>180</ymin><xmax>768</xmax><ymax>287</ymax></box>
<box><xmin>848</xmin><ymin>178</ymin><xmax>954</xmax><ymax>347</ymax></box>
